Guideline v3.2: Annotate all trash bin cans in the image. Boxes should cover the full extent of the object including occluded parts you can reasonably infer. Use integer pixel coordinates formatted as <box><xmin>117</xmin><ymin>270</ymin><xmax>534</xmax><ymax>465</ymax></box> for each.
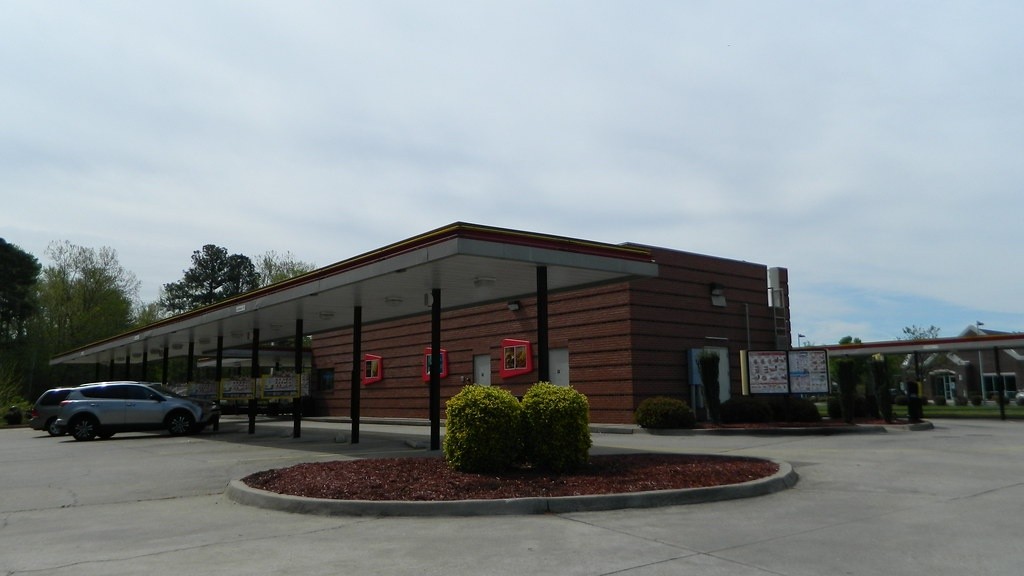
<box><xmin>908</xmin><ymin>394</ymin><xmax>924</xmax><ymax>418</ymax></box>
<box><xmin>9</xmin><ymin>406</ymin><xmax>22</xmax><ymax>425</ymax></box>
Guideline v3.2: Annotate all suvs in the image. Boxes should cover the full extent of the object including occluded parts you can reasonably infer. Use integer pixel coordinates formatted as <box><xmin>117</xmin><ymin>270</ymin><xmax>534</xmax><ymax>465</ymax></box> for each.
<box><xmin>55</xmin><ymin>381</ymin><xmax>221</xmax><ymax>441</ymax></box>
<box><xmin>28</xmin><ymin>387</ymin><xmax>74</xmax><ymax>436</ymax></box>
